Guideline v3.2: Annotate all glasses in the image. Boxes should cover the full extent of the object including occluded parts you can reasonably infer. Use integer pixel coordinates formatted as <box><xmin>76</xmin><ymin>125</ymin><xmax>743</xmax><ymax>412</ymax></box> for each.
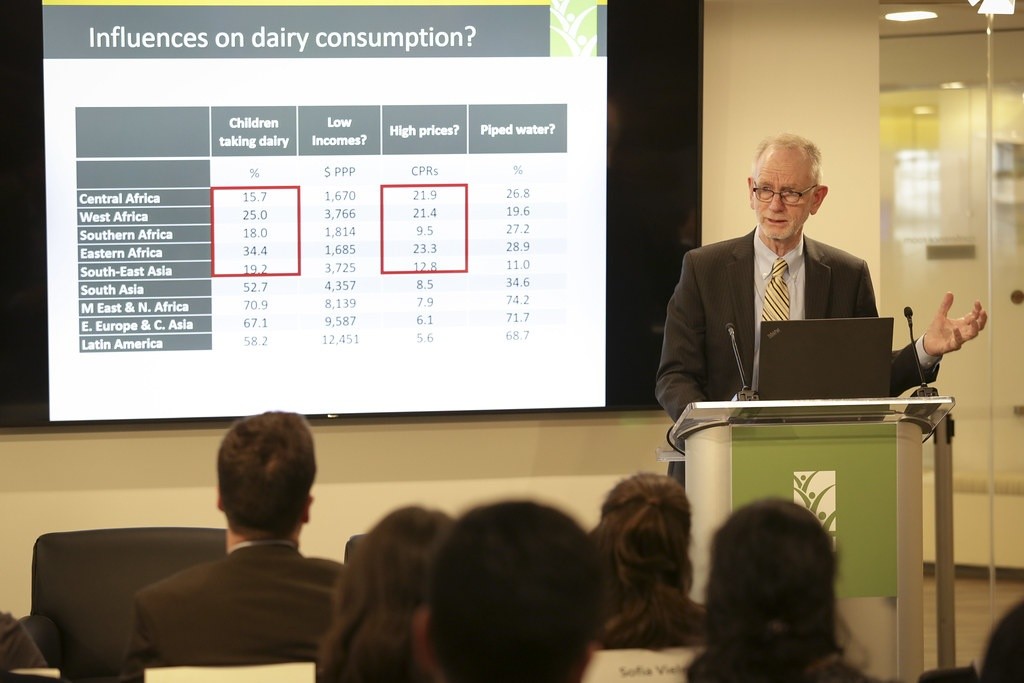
<box><xmin>753</xmin><ymin>180</ymin><xmax>817</xmax><ymax>203</ymax></box>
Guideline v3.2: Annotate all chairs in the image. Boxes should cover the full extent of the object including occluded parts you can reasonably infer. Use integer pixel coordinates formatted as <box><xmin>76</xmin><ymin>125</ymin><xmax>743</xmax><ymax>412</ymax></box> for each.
<box><xmin>29</xmin><ymin>526</ymin><xmax>227</xmax><ymax>683</ymax></box>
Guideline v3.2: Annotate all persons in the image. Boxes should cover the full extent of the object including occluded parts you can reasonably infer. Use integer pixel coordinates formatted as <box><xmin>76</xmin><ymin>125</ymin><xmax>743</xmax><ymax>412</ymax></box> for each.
<box><xmin>1</xmin><ymin>403</ymin><xmax>1024</xmax><ymax>683</ymax></box>
<box><xmin>656</xmin><ymin>134</ymin><xmax>988</xmax><ymax>488</ymax></box>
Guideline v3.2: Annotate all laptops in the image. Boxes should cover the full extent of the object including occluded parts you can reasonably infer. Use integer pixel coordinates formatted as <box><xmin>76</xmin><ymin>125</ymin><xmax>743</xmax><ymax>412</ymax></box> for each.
<box><xmin>750</xmin><ymin>317</ymin><xmax>895</xmax><ymax>424</ymax></box>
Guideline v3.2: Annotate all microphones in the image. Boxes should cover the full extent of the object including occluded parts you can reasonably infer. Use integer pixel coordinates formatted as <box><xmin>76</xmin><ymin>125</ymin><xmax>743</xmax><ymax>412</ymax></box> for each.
<box><xmin>726</xmin><ymin>323</ymin><xmax>758</xmax><ymax>403</ymax></box>
<box><xmin>904</xmin><ymin>306</ymin><xmax>942</xmax><ymax>417</ymax></box>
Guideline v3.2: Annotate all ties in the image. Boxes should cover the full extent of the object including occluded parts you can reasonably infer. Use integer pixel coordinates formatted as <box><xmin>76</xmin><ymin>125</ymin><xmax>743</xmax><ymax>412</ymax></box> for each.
<box><xmin>761</xmin><ymin>258</ymin><xmax>790</xmax><ymax>321</ymax></box>
